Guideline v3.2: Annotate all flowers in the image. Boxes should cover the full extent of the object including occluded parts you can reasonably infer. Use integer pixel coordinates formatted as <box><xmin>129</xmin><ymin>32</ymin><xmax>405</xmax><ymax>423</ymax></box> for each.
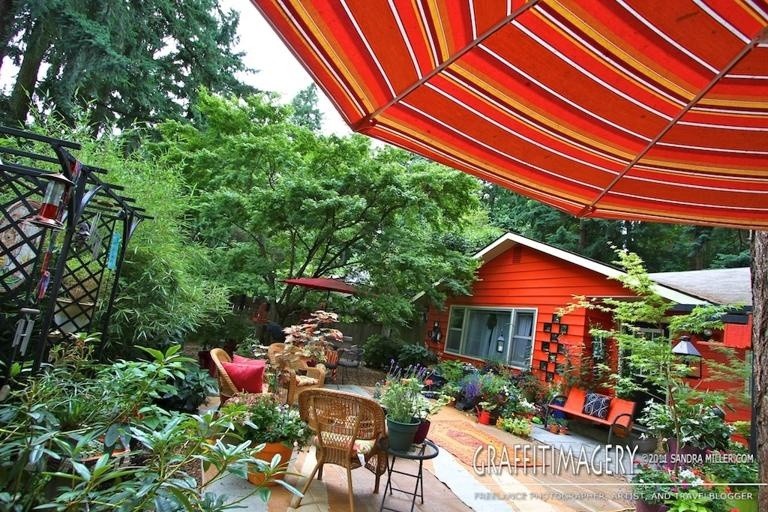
<box><xmin>630</xmin><ymin>459</ymin><xmax>740</xmax><ymax>512</ymax></box>
<box><xmin>379</xmin><ymin>377</ymin><xmax>429</xmax><ymax>423</ymax></box>
<box><xmin>409</xmin><ymin>378</ymin><xmax>456</xmax><ymax>422</ymax></box>
<box><xmin>213</xmin><ymin>389</ymin><xmax>316</xmax><ymax>453</ymax></box>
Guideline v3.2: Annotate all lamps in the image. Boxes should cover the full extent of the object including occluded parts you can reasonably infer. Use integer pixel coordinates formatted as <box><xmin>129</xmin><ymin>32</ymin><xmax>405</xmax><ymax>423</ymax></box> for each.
<box><xmin>495</xmin><ymin>322</ymin><xmax>517</xmax><ymax>353</ymax></box>
<box><xmin>430</xmin><ymin>320</ymin><xmax>440</xmax><ymax>342</ymax></box>
<box><xmin>28</xmin><ymin>166</ymin><xmax>74</xmax><ymax>231</ymax></box>
<box><xmin>670</xmin><ymin>329</ymin><xmax>724</xmax><ymax>357</ymax></box>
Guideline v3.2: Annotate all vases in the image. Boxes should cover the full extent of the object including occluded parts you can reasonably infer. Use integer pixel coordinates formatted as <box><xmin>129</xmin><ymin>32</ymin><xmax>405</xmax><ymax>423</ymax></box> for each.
<box><xmin>387</xmin><ymin>414</ymin><xmax>421</xmax><ymax>453</ymax></box>
<box><xmin>413</xmin><ymin>416</ymin><xmax>430</xmax><ymax>445</ymax></box>
<box><xmin>246</xmin><ymin>436</ymin><xmax>294</xmax><ymax>487</ymax></box>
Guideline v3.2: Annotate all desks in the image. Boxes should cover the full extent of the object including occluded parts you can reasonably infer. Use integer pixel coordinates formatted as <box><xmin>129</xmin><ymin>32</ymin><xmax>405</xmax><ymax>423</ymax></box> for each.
<box><xmin>379</xmin><ymin>435</ymin><xmax>440</xmax><ymax>511</ymax></box>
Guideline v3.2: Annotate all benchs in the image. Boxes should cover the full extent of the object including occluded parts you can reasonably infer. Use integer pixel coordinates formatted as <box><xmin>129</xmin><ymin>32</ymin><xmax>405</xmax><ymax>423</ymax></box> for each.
<box><xmin>543</xmin><ymin>386</ymin><xmax>637</xmax><ymax>453</ymax></box>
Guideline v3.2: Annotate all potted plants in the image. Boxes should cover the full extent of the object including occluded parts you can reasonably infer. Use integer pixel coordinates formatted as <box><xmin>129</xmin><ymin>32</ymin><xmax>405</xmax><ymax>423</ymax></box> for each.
<box><xmin>554</xmin><ymin>239</ymin><xmax>755</xmax><ymax>512</ymax></box>
<box><xmin>637</xmin><ymin>398</ymin><xmax>733</xmax><ymax>470</ymax></box>
<box><xmin>52</xmin><ymin>287</ymin><xmax>93</xmax><ymax>335</ymax></box>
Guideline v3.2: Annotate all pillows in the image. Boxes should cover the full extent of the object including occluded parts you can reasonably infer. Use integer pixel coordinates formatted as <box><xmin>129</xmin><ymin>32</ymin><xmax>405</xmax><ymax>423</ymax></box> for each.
<box><xmin>582</xmin><ymin>388</ymin><xmax>614</xmax><ymax>421</ymax></box>
<box><xmin>312</xmin><ymin>414</ymin><xmax>377</xmax><ymax>458</ymax></box>
<box><xmin>220</xmin><ymin>361</ymin><xmax>264</xmax><ymax>393</ymax></box>
<box><xmin>232</xmin><ymin>354</ymin><xmax>268</xmax><ymax>373</ymax></box>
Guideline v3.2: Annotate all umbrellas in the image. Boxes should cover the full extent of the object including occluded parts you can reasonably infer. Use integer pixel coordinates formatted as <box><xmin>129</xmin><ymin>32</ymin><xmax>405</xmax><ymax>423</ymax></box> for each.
<box><xmin>253</xmin><ymin>1</ymin><xmax>768</xmax><ymax>232</ymax></box>
<box><xmin>278</xmin><ymin>275</ymin><xmax>380</xmax><ymax>329</ymax></box>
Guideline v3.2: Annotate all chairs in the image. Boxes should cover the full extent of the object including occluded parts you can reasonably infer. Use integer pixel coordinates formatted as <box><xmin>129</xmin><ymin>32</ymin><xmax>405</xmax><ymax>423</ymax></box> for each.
<box><xmin>267</xmin><ymin>342</ymin><xmax>326</xmax><ymax>406</ymax></box>
<box><xmin>209</xmin><ymin>347</ymin><xmax>270</xmax><ymax>416</ymax></box>
<box><xmin>326</xmin><ymin>348</ymin><xmax>344</xmax><ymax>383</ymax></box>
<box><xmin>336</xmin><ymin>348</ymin><xmax>365</xmax><ymax>386</ymax></box>
<box><xmin>291</xmin><ymin>386</ymin><xmax>390</xmax><ymax>511</ymax></box>
